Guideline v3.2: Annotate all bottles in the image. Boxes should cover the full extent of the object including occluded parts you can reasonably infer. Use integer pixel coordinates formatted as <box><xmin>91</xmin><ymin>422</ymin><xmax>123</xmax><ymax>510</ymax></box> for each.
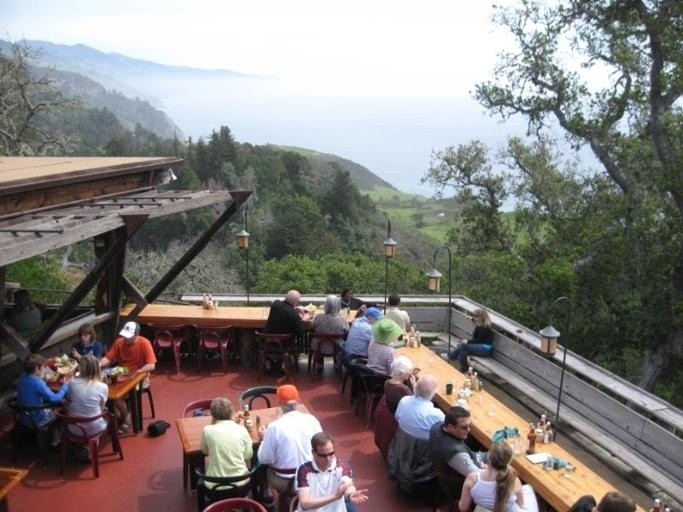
<box><xmin>233</xmin><ymin>403</ymin><xmax>262</xmax><ymax>436</ymax></box>
<box><xmin>456</xmin><ymin>367</ymin><xmax>483</xmax><ymax>410</ymax></box>
<box><xmin>202</xmin><ymin>292</ymin><xmax>217</xmax><ymax>310</ymax></box>
<box><xmin>647</xmin><ymin>495</ymin><xmax>671</xmax><ymax>512</ymax></box>
<box><xmin>404</xmin><ymin>325</ymin><xmax>422</xmax><ymax>349</ymax></box>
<box><xmin>525</xmin><ymin>410</ymin><xmax>554</xmax><ymax>455</ymax></box>
<box><xmin>43</xmin><ymin>354</ymin><xmax>79</xmax><ymax>384</ymax></box>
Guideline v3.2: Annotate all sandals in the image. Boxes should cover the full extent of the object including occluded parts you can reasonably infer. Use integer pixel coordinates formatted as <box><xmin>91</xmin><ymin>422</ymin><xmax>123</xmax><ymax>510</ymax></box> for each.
<box><xmin>119</xmin><ymin>417</ymin><xmax>132</xmax><ymax>434</ymax></box>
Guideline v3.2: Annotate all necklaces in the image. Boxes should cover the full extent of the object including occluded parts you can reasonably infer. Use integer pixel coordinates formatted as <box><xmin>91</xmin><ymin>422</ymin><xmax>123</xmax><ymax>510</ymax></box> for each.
<box><xmin>340</xmin><ymin>298</ymin><xmax>351</xmax><ymax>308</ymax></box>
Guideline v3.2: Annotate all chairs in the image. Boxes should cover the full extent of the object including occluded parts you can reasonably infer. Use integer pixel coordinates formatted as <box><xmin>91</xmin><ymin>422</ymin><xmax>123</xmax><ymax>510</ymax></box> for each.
<box><xmin>10</xmin><ymin>361</ymin><xmax>155</xmax><ymax>479</ymax></box>
<box><xmin>181</xmin><ymin>385</ymin><xmax>355</xmax><ymax>511</ymax></box>
<box><xmin>149</xmin><ymin>326</ymin><xmax>347</xmax><ymax>384</ymax></box>
<box><xmin>339</xmin><ymin>341</ymin><xmax>476</xmax><ymax>511</ymax></box>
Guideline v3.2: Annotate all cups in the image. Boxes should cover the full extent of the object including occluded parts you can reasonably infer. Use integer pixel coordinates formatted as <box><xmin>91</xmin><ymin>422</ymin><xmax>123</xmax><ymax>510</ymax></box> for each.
<box><xmin>446</xmin><ymin>383</ymin><xmax>452</xmax><ymax>394</ymax></box>
<box><xmin>108</xmin><ymin>372</ymin><xmax>118</xmax><ymax>385</ymax></box>
<box><xmin>121</xmin><ymin>363</ymin><xmax>128</xmax><ymax>375</ymax></box>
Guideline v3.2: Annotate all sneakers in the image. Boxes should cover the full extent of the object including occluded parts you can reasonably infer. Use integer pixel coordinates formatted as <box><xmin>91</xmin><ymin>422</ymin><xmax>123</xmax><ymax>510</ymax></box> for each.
<box><xmin>257</xmin><ymin>485</ymin><xmax>275</xmax><ymax>508</ymax></box>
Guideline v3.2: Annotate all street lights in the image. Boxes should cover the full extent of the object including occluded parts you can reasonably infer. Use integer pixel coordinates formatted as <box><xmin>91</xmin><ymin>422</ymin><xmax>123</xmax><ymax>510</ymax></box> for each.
<box><xmin>425</xmin><ymin>246</ymin><xmax>451</xmax><ymax>362</ymax></box>
<box><xmin>235</xmin><ymin>209</ymin><xmax>251</xmax><ymax>305</ymax></box>
<box><xmin>382</xmin><ymin>219</ymin><xmax>397</xmax><ymax>319</ymax></box>
<box><xmin>537</xmin><ymin>296</ymin><xmax>571</xmax><ymax>444</ymax></box>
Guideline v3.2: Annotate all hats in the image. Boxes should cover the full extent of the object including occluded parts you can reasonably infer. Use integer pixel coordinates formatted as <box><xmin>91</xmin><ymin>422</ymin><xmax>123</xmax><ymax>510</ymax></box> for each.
<box><xmin>119</xmin><ymin>321</ymin><xmax>141</xmax><ymax>339</ymax></box>
<box><xmin>365</xmin><ymin>307</ymin><xmax>382</xmax><ymax>320</ymax></box>
<box><xmin>371</xmin><ymin>317</ymin><xmax>404</xmax><ymax>344</ymax></box>
<box><xmin>277</xmin><ymin>384</ymin><xmax>299</xmax><ymax>404</ymax></box>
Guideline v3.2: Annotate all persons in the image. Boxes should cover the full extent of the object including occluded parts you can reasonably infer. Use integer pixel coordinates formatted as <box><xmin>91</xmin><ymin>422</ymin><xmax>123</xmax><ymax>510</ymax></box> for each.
<box><xmin>293</xmin><ymin>430</ymin><xmax>369</xmax><ymax>511</ymax></box>
<box><xmin>253</xmin><ymin>384</ymin><xmax>325</xmax><ymax>505</ymax></box>
<box><xmin>379</xmin><ymin>294</ymin><xmax>410</xmax><ymax>330</ymax></box>
<box><xmin>15</xmin><ymin>352</ymin><xmax>69</xmax><ymax>446</ymax></box>
<box><xmin>309</xmin><ymin>294</ymin><xmax>351</xmax><ymax>375</ymax></box>
<box><xmin>260</xmin><ymin>290</ymin><xmax>312</xmax><ymax>379</ymax></box>
<box><xmin>64</xmin><ymin>355</ymin><xmax>111</xmax><ymax>464</ymax></box>
<box><xmin>198</xmin><ymin>395</ymin><xmax>256</xmax><ymax>499</ymax></box>
<box><xmin>69</xmin><ymin>323</ymin><xmax>102</xmax><ymax>363</ymax></box>
<box><xmin>365</xmin><ymin>318</ymin><xmax>405</xmax><ymax>379</ymax></box>
<box><xmin>339</xmin><ymin>286</ymin><xmax>379</xmax><ymax>309</ymax></box>
<box><xmin>7</xmin><ymin>289</ymin><xmax>47</xmax><ymax>338</ymax></box>
<box><xmin>97</xmin><ymin>320</ymin><xmax>158</xmax><ymax>436</ymax></box>
<box><xmin>376</xmin><ymin>354</ymin><xmax>420</xmax><ymax>447</ymax></box>
<box><xmin>428</xmin><ymin>406</ymin><xmax>488</xmax><ymax>481</ymax></box>
<box><xmin>341</xmin><ymin>306</ymin><xmax>381</xmax><ymax>364</ymax></box>
<box><xmin>458</xmin><ymin>439</ymin><xmax>525</xmax><ymax>511</ymax></box>
<box><xmin>567</xmin><ymin>489</ymin><xmax>636</xmax><ymax>511</ymax></box>
<box><xmin>383</xmin><ymin>377</ymin><xmax>448</xmax><ymax>486</ymax></box>
<box><xmin>439</xmin><ymin>308</ymin><xmax>494</xmax><ymax>372</ymax></box>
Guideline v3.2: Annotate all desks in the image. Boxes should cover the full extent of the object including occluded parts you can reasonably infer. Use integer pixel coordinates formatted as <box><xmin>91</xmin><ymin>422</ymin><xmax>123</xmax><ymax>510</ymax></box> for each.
<box><xmin>175</xmin><ymin>404</ymin><xmax>310</xmax><ymax>511</ymax></box>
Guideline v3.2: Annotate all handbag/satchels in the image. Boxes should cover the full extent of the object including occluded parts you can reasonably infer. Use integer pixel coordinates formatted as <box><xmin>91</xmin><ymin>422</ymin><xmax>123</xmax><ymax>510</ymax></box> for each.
<box><xmin>147</xmin><ymin>420</ymin><xmax>171</xmax><ymax>435</ymax></box>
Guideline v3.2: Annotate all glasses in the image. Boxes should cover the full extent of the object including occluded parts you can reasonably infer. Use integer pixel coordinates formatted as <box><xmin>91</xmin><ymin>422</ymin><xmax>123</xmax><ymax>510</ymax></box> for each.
<box><xmin>316</xmin><ymin>449</ymin><xmax>335</xmax><ymax>457</ymax></box>
<box><xmin>460</xmin><ymin>424</ymin><xmax>472</xmax><ymax>430</ymax></box>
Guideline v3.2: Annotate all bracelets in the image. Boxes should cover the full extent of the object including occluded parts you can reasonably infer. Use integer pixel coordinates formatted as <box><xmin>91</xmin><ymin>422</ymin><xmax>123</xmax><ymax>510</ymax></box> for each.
<box><xmin>347</xmin><ymin>490</ymin><xmax>353</xmax><ymax>501</ymax></box>
